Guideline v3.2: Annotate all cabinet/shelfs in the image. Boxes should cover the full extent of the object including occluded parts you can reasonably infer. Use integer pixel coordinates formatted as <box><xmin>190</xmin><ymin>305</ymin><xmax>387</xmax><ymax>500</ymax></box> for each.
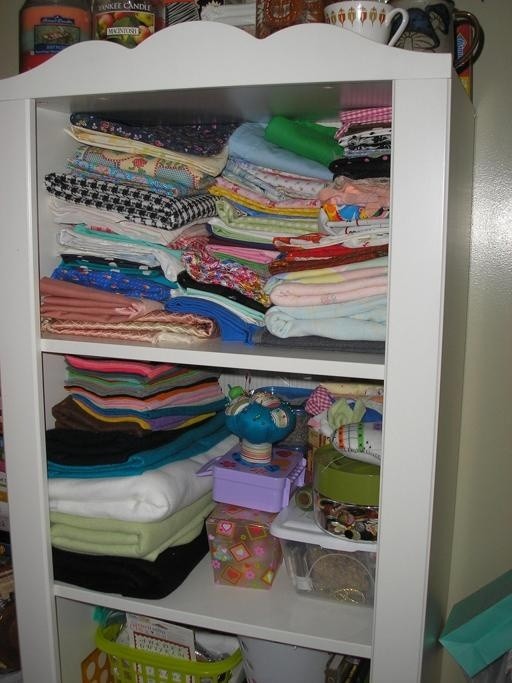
<box><xmin>0</xmin><ymin>21</ymin><xmax>474</xmax><ymax>683</ymax></box>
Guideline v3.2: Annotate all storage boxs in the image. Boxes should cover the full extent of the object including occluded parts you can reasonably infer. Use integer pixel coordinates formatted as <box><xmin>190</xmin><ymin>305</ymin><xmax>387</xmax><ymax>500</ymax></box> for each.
<box><xmin>270</xmin><ymin>492</ymin><xmax>377</xmax><ymax>606</ymax></box>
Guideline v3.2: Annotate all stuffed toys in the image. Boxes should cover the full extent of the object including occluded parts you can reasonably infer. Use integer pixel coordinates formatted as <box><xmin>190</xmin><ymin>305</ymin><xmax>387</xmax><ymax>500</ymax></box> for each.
<box><xmin>223</xmin><ymin>382</ymin><xmax>296</xmax><ymax>465</ymax></box>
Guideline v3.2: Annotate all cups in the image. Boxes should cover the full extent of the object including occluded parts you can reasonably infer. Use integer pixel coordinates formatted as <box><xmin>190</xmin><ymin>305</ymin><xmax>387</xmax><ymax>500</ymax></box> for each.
<box><xmin>323</xmin><ymin>0</ymin><xmax>410</xmax><ymax>45</ymax></box>
